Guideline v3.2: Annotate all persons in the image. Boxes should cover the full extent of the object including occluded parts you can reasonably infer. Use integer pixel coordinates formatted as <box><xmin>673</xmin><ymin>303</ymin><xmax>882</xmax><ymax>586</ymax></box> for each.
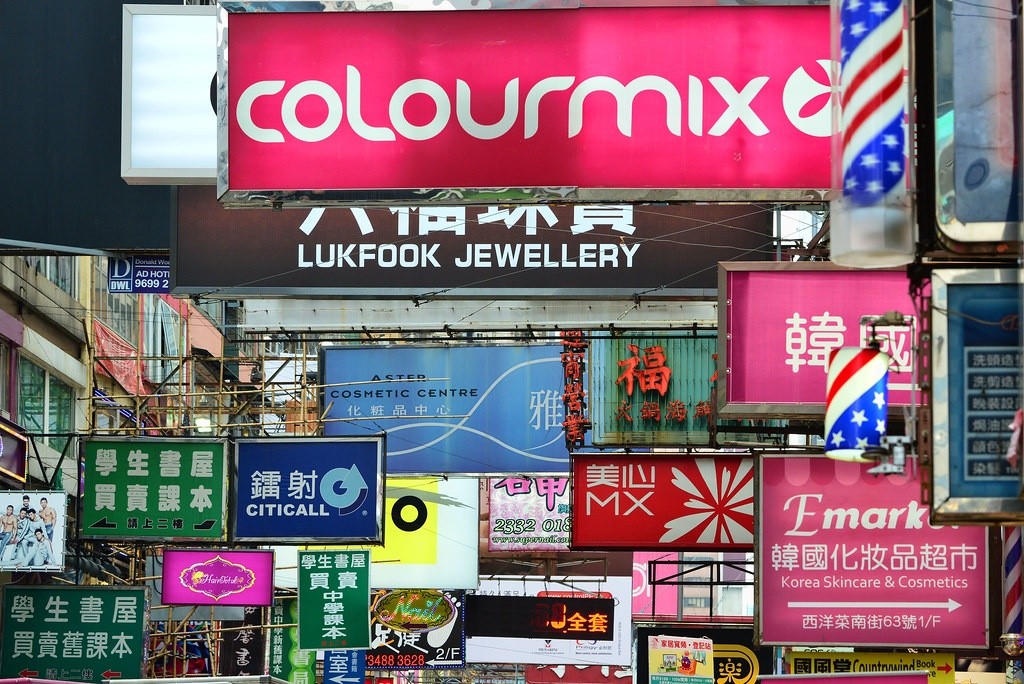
<box><xmin>0</xmin><ymin>495</ymin><xmax>62</xmax><ymax>568</ymax></box>
